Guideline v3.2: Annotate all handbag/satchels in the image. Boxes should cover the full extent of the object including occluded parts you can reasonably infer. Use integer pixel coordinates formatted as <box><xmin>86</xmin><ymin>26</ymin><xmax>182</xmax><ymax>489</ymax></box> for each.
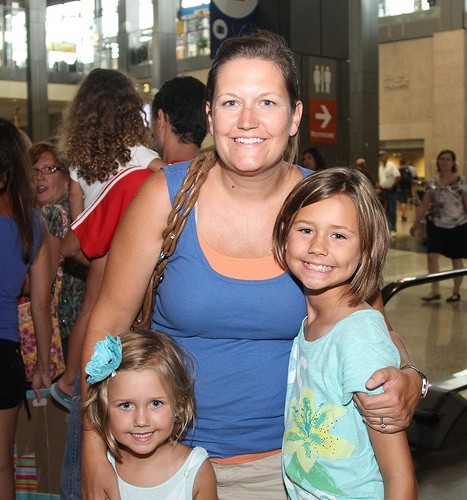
<box><xmin>18</xmin><ymin>297</ymin><xmax>66</xmax><ymax>384</ymax></box>
<box><xmin>131</xmin><ymin>150</ymin><xmax>220</xmax><ymax>330</ymax></box>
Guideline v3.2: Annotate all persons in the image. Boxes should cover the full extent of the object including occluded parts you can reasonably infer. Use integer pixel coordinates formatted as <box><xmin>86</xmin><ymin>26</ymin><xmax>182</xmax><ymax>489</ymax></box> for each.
<box><xmin>0</xmin><ymin>118</ymin><xmax>54</xmax><ymax>500</ymax></box>
<box><xmin>356</xmin><ymin>158</ymin><xmax>376</xmax><ymax>187</ymax></box>
<box><xmin>271</xmin><ymin>166</ymin><xmax>420</xmax><ymax>500</ymax></box>
<box><xmin>399</xmin><ymin>159</ymin><xmax>413</xmax><ymax>221</ymax></box>
<box><xmin>81</xmin><ymin>329</ymin><xmax>219</xmax><ymax>500</ymax></box>
<box><xmin>59</xmin><ymin>76</ymin><xmax>207</xmax><ymax>499</ymax></box>
<box><xmin>49</xmin><ymin>68</ymin><xmax>172</xmax><ymax>411</ymax></box>
<box><xmin>82</xmin><ymin>29</ymin><xmax>429</xmax><ymax>500</ymax></box>
<box><xmin>303</xmin><ymin>146</ymin><xmax>325</xmax><ymax>172</ymax></box>
<box><xmin>17</xmin><ymin>136</ymin><xmax>89</xmax><ymax>500</ymax></box>
<box><xmin>376</xmin><ymin>151</ymin><xmax>401</xmax><ymax>239</ymax></box>
<box><xmin>410</xmin><ymin>150</ymin><xmax>467</xmax><ymax>303</ymax></box>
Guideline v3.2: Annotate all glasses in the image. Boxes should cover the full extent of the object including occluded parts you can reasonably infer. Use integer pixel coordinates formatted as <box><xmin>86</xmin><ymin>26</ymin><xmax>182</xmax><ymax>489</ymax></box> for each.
<box><xmin>30</xmin><ymin>166</ymin><xmax>65</xmax><ymax>177</ymax></box>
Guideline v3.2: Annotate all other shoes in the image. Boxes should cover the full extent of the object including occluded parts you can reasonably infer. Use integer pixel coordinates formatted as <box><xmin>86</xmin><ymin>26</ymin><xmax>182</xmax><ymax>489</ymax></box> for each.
<box><xmin>391</xmin><ymin>231</ymin><xmax>396</xmax><ymax>238</ymax></box>
<box><xmin>402</xmin><ymin>217</ymin><xmax>406</xmax><ymax>221</ymax></box>
<box><xmin>420</xmin><ymin>294</ymin><xmax>440</xmax><ymax>301</ymax></box>
<box><xmin>445</xmin><ymin>294</ymin><xmax>461</xmax><ymax>302</ymax></box>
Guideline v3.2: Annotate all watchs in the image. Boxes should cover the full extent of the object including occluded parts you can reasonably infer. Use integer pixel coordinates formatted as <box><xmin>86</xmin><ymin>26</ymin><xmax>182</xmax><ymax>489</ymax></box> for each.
<box><xmin>399</xmin><ymin>364</ymin><xmax>429</xmax><ymax>399</ymax></box>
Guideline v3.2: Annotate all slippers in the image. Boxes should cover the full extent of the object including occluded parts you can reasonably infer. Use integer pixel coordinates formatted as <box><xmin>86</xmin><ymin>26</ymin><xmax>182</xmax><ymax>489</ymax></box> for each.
<box><xmin>50</xmin><ymin>381</ymin><xmax>73</xmax><ymax>412</ymax></box>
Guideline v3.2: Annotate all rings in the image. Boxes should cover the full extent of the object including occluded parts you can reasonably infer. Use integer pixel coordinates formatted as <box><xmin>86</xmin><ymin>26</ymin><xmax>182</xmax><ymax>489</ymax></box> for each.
<box><xmin>380</xmin><ymin>417</ymin><xmax>385</xmax><ymax>428</ymax></box>
